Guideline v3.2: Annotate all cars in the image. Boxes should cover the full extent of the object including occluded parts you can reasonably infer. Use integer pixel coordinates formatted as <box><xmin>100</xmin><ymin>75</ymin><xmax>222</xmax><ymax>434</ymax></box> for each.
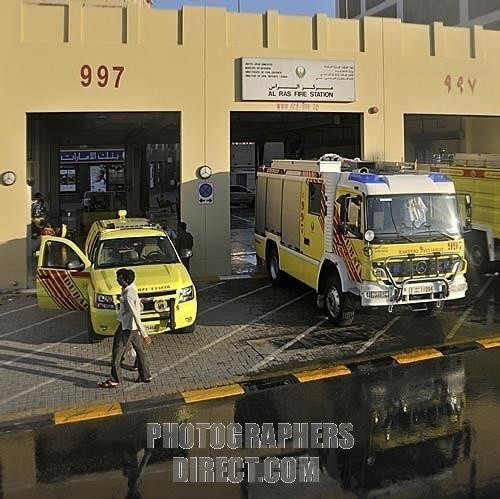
<box><xmin>36</xmin><ymin>209</ymin><xmax>198</xmax><ymax>336</ymax></box>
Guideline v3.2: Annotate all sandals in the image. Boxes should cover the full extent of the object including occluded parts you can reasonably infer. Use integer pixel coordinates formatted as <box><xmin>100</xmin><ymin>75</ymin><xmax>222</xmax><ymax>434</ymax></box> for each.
<box><xmin>134</xmin><ymin>377</ymin><xmax>151</xmax><ymax>383</ymax></box>
<box><xmin>98</xmin><ymin>379</ymin><xmax>118</xmax><ymax>387</ymax></box>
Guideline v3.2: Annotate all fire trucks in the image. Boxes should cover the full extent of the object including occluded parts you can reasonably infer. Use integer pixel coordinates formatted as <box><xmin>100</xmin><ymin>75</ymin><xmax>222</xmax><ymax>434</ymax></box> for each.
<box><xmin>234</xmin><ymin>354</ymin><xmax>469</xmax><ymax>498</ymax></box>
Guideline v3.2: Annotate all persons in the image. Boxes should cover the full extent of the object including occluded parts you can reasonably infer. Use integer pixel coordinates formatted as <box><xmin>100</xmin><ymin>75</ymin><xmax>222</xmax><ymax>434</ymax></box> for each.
<box><xmin>161</xmin><ymin>220</ymin><xmax>177</xmax><ymax>245</ymax></box>
<box><xmin>111</xmin><ymin>269</ymin><xmax>145</xmax><ymax>371</ymax></box>
<box><xmin>99</xmin><ymin>268</ymin><xmax>152</xmax><ymax>389</ymax></box>
<box><xmin>121</xmin><ymin>444</ymin><xmax>153</xmax><ymax>498</ymax></box>
<box><xmin>176</xmin><ymin>222</ymin><xmax>194</xmax><ymax>272</ymax></box>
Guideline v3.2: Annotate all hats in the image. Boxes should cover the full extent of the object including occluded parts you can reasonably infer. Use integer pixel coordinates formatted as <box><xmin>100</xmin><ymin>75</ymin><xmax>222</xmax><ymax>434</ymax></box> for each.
<box><xmin>116</xmin><ymin>269</ymin><xmax>136</xmax><ymax>285</ymax></box>
